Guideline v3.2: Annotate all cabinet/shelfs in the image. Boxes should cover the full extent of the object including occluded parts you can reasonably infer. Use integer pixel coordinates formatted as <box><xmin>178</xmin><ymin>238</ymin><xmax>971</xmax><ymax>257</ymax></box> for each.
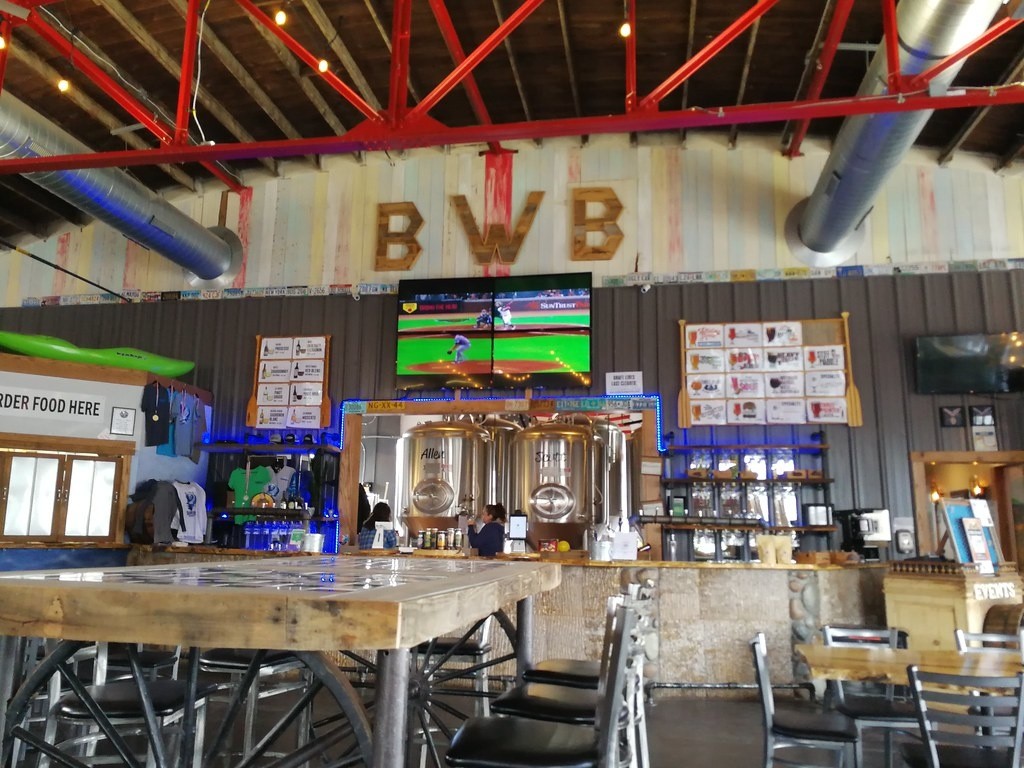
<box><xmin>661</xmin><ymin>442</ymin><xmax>838</xmax><ymax>561</ymax></box>
<box><xmin>203</xmin><ymin>442</ymin><xmax>341</xmax><ymax>542</ymax></box>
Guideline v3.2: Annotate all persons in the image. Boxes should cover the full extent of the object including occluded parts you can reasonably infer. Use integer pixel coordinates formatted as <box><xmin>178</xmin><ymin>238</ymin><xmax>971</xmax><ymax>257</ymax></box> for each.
<box><xmin>420</xmin><ymin>287</ymin><xmax>588</xmax><ymax>362</ymax></box>
<box><xmin>468</xmin><ymin>503</ymin><xmax>507</xmax><ymax>558</ymax></box>
<box><xmin>356</xmin><ymin>482</ymin><xmax>397</xmax><ymax>550</ymax></box>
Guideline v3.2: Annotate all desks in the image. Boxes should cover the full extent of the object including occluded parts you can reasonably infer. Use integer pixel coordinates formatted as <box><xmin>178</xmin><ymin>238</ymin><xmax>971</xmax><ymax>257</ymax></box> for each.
<box><xmin>0</xmin><ymin>554</ymin><xmax>562</xmax><ymax>768</ymax></box>
<box><xmin>794</xmin><ymin>644</ymin><xmax>1024</xmax><ymax>750</ymax></box>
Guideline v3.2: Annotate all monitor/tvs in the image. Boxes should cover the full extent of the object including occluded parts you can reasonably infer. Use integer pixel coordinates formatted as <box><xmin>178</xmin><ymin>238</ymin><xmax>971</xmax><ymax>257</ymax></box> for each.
<box><xmin>394</xmin><ymin>273</ymin><xmax>592</xmax><ymax>391</ymax></box>
<box><xmin>916</xmin><ymin>333</ymin><xmax>1024</xmax><ymax>395</ymax></box>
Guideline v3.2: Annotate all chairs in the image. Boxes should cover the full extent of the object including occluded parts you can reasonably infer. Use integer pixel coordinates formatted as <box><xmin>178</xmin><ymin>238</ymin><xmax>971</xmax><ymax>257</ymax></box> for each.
<box><xmin>906</xmin><ymin>665</ymin><xmax>1024</xmax><ymax>768</ymax></box>
<box><xmin>410</xmin><ymin>616</ymin><xmax>492</xmax><ymax>725</ymax></box>
<box><xmin>445</xmin><ymin>566</ymin><xmax>660</xmax><ymax>768</ymax></box>
<box><xmin>9</xmin><ymin>631</ymin><xmax>313</xmax><ymax>768</ymax></box>
<box><xmin>823</xmin><ymin>626</ymin><xmax>938</xmax><ymax>768</ymax></box>
<box><xmin>954</xmin><ymin>629</ymin><xmax>1024</xmax><ymax>750</ymax></box>
<box><xmin>749</xmin><ymin>632</ymin><xmax>860</xmax><ymax>768</ymax></box>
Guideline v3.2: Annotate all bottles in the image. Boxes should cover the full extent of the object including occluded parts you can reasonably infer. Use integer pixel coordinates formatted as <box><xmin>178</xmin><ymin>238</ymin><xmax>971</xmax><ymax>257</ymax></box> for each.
<box><xmin>418</xmin><ymin>528</ymin><xmax>463</xmax><ymax>550</ymax></box>
<box><xmin>280</xmin><ymin>491</ymin><xmax>303</xmax><ymax>510</ymax></box>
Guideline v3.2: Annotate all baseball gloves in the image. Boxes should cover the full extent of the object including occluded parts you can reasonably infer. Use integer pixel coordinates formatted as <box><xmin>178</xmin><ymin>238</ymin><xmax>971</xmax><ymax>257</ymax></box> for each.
<box><xmin>447</xmin><ymin>350</ymin><xmax>453</xmax><ymax>354</ymax></box>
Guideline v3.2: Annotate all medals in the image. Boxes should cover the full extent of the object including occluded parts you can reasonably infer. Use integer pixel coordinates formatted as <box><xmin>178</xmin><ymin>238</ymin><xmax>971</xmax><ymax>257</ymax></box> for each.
<box><xmin>950</xmin><ymin>417</ymin><xmax>957</xmax><ymax>425</ymax></box>
<box><xmin>152</xmin><ymin>415</ymin><xmax>159</xmax><ymax>421</ymax></box>
<box><xmin>973</xmin><ymin>415</ymin><xmax>993</xmax><ymax>426</ymax></box>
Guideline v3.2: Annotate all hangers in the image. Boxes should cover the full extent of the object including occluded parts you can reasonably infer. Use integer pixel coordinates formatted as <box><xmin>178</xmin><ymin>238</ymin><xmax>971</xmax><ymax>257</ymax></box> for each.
<box><xmin>152</xmin><ymin>377</ymin><xmax>161</xmax><ymax>387</ymax></box>
<box><xmin>168</xmin><ymin>382</ymin><xmax>176</xmax><ymax>392</ymax></box>
<box><xmin>270</xmin><ymin>456</ymin><xmax>284</xmax><ymax>469</ymax></box>
<box><xmin>195</xmin><ymin>391</ymin><xmax>200</xmax><ymax>398</ymax></box>
